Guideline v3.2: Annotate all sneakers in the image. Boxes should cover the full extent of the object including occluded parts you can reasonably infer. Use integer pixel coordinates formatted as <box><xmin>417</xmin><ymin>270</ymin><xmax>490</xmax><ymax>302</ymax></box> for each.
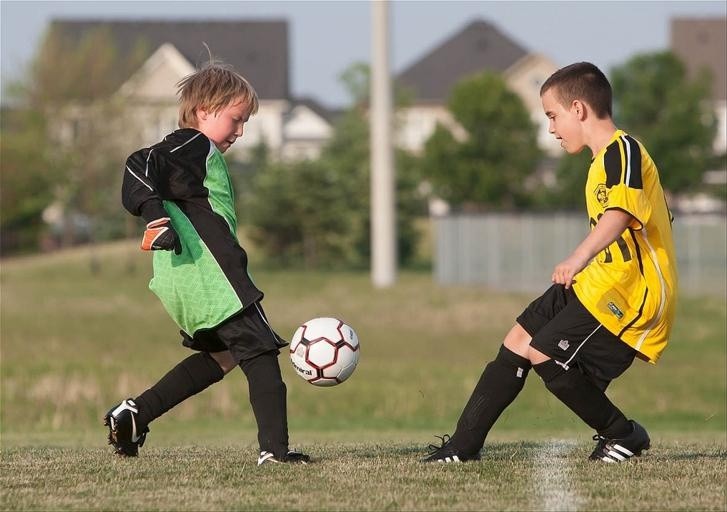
<box><xmin>257</xmin><ymin>448</ymin><xmax>311</xmax><ymax>470</ymax></box>
<box><xmin>418</xmin><ymin>440</ymin><xmax>481</xmax><ymax>464</ymax></box>
<box><xmin>105</xmin><ymin>397</ymin><xmax>149</xmax><ymax>456</ymax></box>
<box><xmin>588</xmin><ymin>419</ymin><xmax>652</xmax><ymax>463</ymax></box>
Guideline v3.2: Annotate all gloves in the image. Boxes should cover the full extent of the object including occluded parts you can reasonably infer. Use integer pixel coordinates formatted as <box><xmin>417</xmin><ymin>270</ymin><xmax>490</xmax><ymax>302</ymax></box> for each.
<box><xmin>141</xmin><ymin>216</ymin><xmax>182</xmax><ymax>256</ymax></box>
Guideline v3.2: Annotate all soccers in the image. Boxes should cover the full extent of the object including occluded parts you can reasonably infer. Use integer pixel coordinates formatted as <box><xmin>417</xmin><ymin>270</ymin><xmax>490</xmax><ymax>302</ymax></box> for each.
<box><xmin>290</xmin><ymin>317</ymin><xmax>360</xmax><ymax>386</ymax></box>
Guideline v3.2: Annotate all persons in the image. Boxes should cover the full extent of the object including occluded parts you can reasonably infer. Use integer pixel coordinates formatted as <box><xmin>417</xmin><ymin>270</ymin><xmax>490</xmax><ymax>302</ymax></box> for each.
<box><xmin>414</xmin><ymin>60</ymin><xmax>682</xmax><ymax>468</ymax></box>
<box><xmin>98</xmin><ymin>41</ymin><xmax>314</xmax><ymax>469</ymax></box>
